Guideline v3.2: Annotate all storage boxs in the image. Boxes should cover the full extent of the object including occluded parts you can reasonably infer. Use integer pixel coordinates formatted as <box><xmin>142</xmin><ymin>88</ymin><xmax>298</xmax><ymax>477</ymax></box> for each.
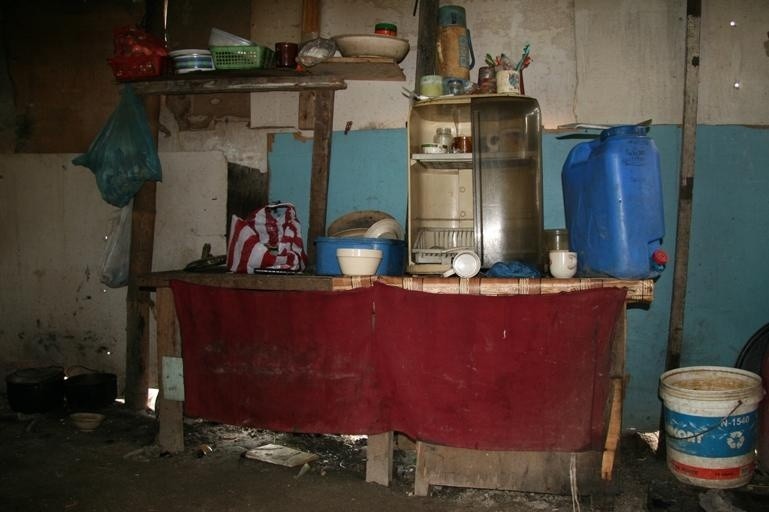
<box><xmin>315</xmin><ymin>236</ymin><xmax>407</xmax><ymax>276</ymax></box>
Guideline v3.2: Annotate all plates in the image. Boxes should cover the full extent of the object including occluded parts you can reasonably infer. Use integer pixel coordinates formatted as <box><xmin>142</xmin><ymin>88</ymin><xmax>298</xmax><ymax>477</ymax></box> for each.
<box><xmin>326</xmin><ymin>210</ymin><xmax>402</xmax><ymax>240</ymax></box>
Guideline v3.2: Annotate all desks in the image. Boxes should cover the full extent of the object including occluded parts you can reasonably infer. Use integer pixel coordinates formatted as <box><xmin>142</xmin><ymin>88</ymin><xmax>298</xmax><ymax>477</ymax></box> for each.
<box><xmin>140</xmin><ymin>271</ymin><xmax>654</xmax><ymax>483</ymax></box>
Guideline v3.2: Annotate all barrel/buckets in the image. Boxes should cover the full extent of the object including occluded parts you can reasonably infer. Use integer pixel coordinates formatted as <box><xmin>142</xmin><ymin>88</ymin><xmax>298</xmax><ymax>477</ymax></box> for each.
<box><xmin>658</xmin><ymin>366</ymin><xmax>766</xmax><ymax>490</ymax></box>
<box><xmin>561</xmin><ymin>125</ymin><xmax>669</xmax><ymax>281</ymax></box>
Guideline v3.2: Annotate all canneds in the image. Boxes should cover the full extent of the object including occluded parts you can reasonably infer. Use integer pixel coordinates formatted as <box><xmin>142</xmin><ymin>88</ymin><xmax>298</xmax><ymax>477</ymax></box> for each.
<box><xmin>447</xmin><ymin>79</ymin><xmax>464</xmax><ymax>95</ymax></box>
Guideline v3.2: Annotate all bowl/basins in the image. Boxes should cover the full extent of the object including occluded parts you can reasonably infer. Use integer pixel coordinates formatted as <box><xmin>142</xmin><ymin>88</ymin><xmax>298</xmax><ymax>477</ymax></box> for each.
<box><xmin>420</xmin><ymin>143</ymin><xmax>449</xmax><ymax>155</ymax></box>
<box><xmin>336</xmin><ymin>247</ymin><xmax>383</xmax><ymax>276</ymax></box>
<box><xmin>172</xmin><ymin>53</ymin><xmax>216</xmax><ymax>75</ymax></box>
<box><xmin>331</xmin><ymin>34</ymin><xmax>411</xmax><ymax>61</ymax></box>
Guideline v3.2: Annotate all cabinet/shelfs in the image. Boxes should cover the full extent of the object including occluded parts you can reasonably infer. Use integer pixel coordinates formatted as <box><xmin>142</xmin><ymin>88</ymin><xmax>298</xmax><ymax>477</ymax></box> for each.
<box><xmin>407</xmin><ymin>94</ymin><xmax>544</xmax><ymax>275</ymax></box>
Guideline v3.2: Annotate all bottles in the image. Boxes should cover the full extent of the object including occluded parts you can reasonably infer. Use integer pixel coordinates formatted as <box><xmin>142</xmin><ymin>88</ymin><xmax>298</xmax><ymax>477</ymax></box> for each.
<box><xmin>478</xmin><ymin>67</ymin><xmax>496</xmax><ymax>91</ymax></box>
<box><xmin>420</xmin><ymin>75</ymin><xmax>444</xmax><ymax>96</ymax></box>
<box><xmin>433</xmin><ymin>127</ymin><xmax>471</xmax><ymax>154</ymax></box>
<box><xmin>448</xmin><ymin>79</ymin><xmax>465</xmax><ymax>96</ymax></box>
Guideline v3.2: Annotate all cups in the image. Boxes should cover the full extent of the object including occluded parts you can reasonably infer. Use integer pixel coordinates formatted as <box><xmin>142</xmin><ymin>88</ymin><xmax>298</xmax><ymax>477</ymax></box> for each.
<box><xmin>495</xmin><ymin>70</ymin><xmax>521</xmax><ymax>93</ymax></box>
<box><xmin>442</xmin><ymin>250</ymin><xmax>481</xmax><ymax>279</ymax></box>
<box><xmin>547</xmin><ymin>250</ymin><xmax>578</xmax><ymax>280</ymax></box>
